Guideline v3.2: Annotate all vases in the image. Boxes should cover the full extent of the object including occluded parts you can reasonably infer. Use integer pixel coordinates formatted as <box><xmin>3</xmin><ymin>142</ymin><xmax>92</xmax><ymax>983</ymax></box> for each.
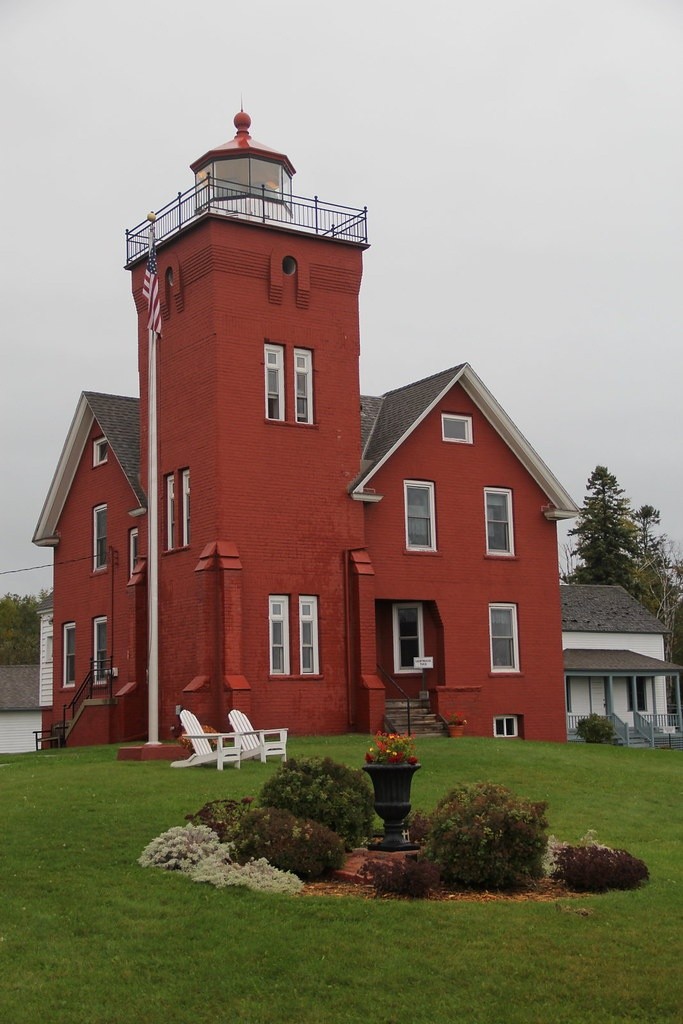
<box><xmin>361</xmin><ymin>764</ymin><xmax>422</xmax><ymax>853</ymax></box>
<box><xmin>448</xmin><ymin>726</ymin><xmax>465</xmax><ymax>737</ymax></box>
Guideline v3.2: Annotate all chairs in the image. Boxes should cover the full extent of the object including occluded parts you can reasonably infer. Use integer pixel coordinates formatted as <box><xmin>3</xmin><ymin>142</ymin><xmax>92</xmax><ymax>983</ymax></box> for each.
<box><xmin>169</xmin><ymin>710</ymin><xmax>244</xmax><ymax>771</ymax></box>
<box><xmin>228</xmin><ymin>710</ymin><xmax>289</xmax><ymax>764</ymax></box>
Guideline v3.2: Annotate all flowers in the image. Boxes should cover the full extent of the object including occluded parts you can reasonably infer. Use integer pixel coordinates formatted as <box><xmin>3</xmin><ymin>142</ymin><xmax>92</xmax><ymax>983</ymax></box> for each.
<box><xmin>365</xmin><ymin>729</ymin><xmax>417</xmax><ymax>764</ymax></box>
<box><xmin>444</xmin><ymin>711</ymin><xmax>467</xmax><ymax>725</ymax></box>
<box><xmin>176</xmin><ymin>725</ymin><xmax>224</xmax><ymax>753</ymax></box>
